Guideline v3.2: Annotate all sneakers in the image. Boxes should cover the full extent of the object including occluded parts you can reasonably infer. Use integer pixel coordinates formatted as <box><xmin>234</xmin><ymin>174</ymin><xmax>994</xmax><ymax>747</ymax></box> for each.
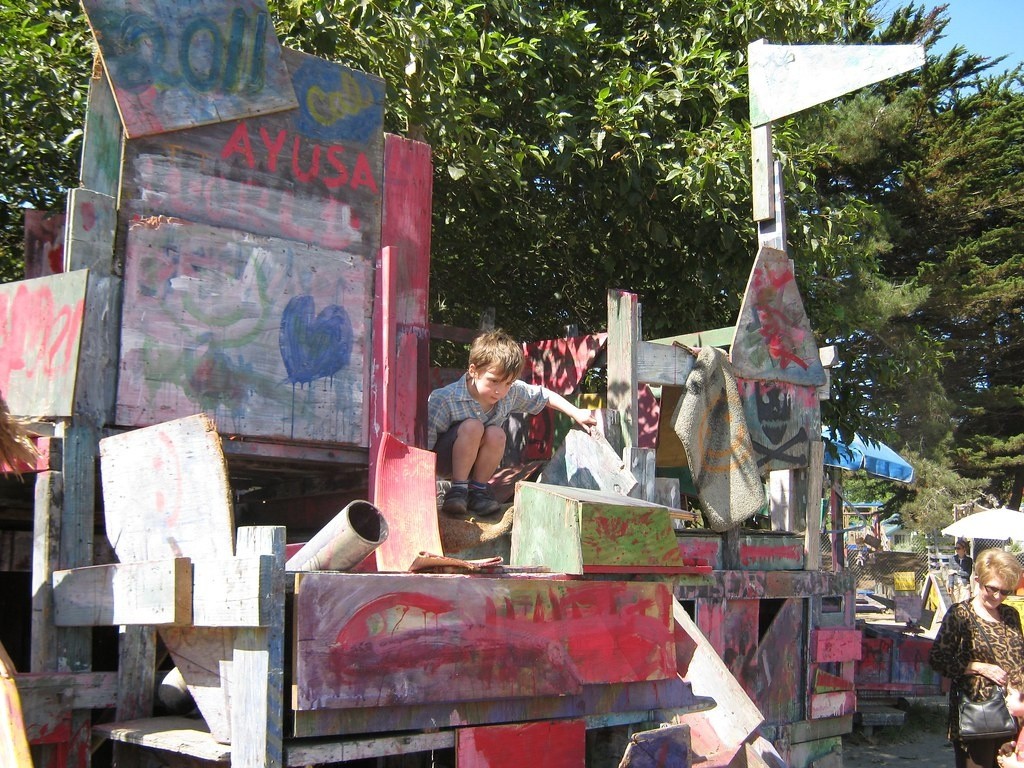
<box><xmin>442</xmin><ymin>486</ymin><xmax>468</xmax><ymax>514</ymax></box>
<box><xmin>468</xmin><ymin>489</ymin><xmax>500</xmax><ymax>516</ymax></box>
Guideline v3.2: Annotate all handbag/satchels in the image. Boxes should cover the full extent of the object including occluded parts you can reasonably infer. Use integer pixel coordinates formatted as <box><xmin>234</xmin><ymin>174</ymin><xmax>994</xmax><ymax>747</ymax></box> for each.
<box><xmin>957</xmin><ymin>687</ymin><xmax>1017</xmax><ymax>740</ymax></box>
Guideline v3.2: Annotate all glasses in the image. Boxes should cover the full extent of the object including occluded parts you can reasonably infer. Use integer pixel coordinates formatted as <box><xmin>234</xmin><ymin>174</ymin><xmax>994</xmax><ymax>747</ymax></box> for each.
<box><xmin>954</xmin><ymin>547</ymin><xmax>963</xmax><ymax>550</ymax></box>
<box><xmin>979</xmin><ymin>579</ymin><xmax>1014</xmax><ymax>595</ymax></box>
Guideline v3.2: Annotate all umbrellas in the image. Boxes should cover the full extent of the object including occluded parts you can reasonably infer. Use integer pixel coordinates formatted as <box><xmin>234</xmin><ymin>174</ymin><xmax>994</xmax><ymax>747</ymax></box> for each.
<box><xmin>941</xmin><ymin>508</ymin><xmax>1024</xmax><ymax>552</ymax></box>
<box><xmin>821</xmin><ymin>425</ymin><xmax>915</xmax><ymax>483</ymax></box>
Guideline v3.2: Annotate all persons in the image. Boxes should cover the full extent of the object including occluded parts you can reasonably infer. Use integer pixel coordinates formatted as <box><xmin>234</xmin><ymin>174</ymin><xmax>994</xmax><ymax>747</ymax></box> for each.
<box><xmin>997</xmin><ymin>674</ymin><xmax>1024</xmax><ymax>768</ymax></box>
<box><xmin>946</xmin><ymin>541</ymin><xmax>973</xmax><ymax>606</ymax></box>
<box><xmin>927</xmin><ymin>547</ymin><xmax>1024</xmax><ymax>768</ymax></box>
<box><xmin>427</xmin><ymin>330</ymin><xmax>598</xmax><ymax>515</ymax></box>
